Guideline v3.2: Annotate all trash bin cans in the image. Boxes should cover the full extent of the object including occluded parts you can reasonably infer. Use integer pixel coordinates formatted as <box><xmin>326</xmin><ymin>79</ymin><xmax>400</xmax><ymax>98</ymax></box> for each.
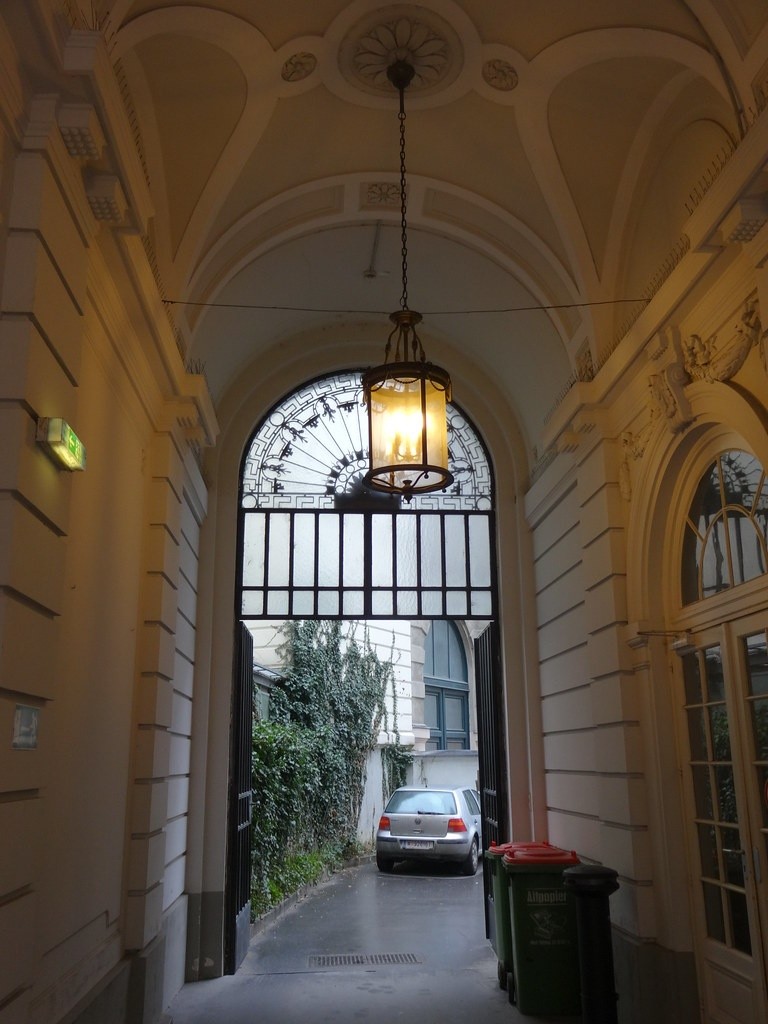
<box><xmin>484</xmin><ymin>837</ymin><xmax>580</xmax><ymax>1017</ymax></box>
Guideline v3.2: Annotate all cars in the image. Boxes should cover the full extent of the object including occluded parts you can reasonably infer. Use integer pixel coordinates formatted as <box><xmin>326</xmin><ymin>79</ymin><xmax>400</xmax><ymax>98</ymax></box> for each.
<box><xmin>375</xmin><ymin>786</ymin><xmax>483</xmax><ymax>877</ymax></box>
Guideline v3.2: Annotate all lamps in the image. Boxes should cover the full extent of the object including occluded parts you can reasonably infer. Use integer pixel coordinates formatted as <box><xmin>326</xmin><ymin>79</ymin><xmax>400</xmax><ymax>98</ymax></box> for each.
<box><xmin>359</xmin><ymin>61</ymin><xmax>452</xmax><ymax>505</ymax></box>
